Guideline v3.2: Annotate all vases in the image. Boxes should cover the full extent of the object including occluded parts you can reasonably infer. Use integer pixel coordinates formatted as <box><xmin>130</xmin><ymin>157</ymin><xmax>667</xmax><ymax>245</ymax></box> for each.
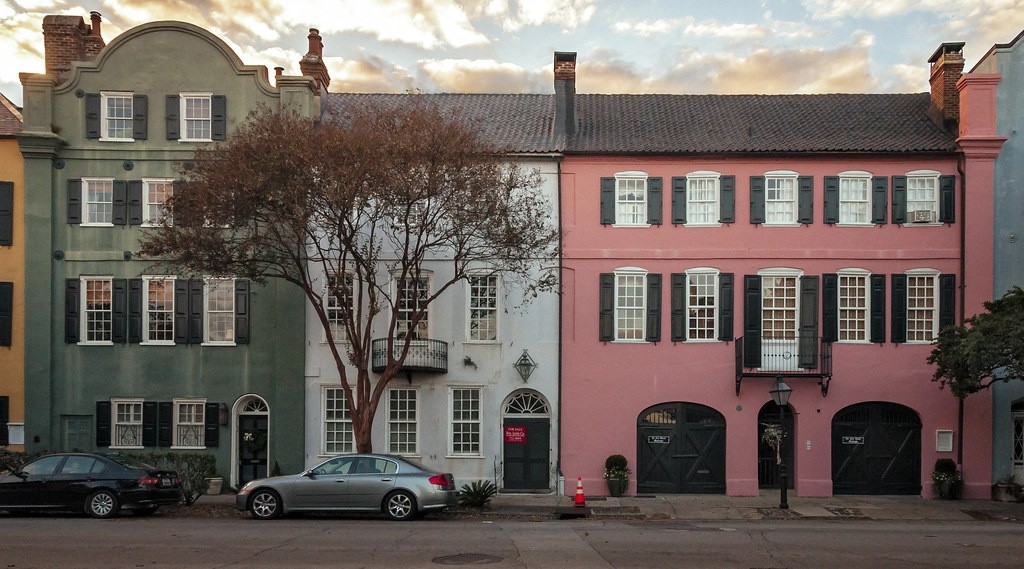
<box><xmin>940</xmin><ymin>486</ymin><xmax>955</xmax><ymax>499</ymax></box>
<box><xmin>608</xmin><ymin>478</ymin><xmax>622</xmax><ymax>497</ymax></box>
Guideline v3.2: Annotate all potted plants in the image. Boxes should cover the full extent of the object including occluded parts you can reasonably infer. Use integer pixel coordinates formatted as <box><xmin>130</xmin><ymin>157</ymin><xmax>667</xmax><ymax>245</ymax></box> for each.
<box><xmin>204</xmin><ymin>458</ymin><xmax>223</xmax><ymax>494</ymax></box>
<box><xmin>995</xmin><ymin>473</ymin><xmax>1016</xmax><ymax>502</ymax></box>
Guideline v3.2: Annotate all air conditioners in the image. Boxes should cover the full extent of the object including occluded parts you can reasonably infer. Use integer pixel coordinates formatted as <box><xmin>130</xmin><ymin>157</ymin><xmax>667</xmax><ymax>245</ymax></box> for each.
<box><xmin>911</xmin><ymin>210</ymin><xmax>932</xmax><ymax>222</ymax></box>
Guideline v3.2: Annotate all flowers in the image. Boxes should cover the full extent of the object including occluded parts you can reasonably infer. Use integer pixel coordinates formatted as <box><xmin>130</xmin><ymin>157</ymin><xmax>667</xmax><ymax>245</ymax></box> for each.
<box><xmin>604</xmin><ymin>454</ymin><xmax>632</xmax><ymax>495</ymax></box>
<box><xmin>931</xmin><ymin>458</ymin><xmax>961</xmax><ymax>498</ymax></box>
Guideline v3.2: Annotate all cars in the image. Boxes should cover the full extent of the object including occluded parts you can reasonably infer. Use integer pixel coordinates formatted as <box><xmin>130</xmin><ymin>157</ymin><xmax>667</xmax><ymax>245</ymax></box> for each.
<box><xmin>236</xmin><ymin>453</ymin><xmax>460</xmax><ymax>521</ymax></box>
<box><xmin>0</xmin><ymin>451</ymin><xmax>184</xmax><ymax>519</ymax></box>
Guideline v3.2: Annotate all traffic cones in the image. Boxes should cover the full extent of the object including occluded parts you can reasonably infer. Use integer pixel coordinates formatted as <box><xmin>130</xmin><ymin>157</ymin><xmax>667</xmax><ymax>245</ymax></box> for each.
<box><xmin>574</xmin><ymin>477</ymin><xmax>586</xmax><ymax>504</ymax></box>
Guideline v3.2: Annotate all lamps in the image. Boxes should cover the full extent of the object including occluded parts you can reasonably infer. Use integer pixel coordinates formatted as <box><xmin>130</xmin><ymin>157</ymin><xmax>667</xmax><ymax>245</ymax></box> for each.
<box><xmin>219</xmin><ymin>402</ymin><xmax>228</xmax><ymax>424</ymax></box>
<box><xmin>512</xmin><ymin>349</ymin><xmax>538</xmax><ymax>382</ymax></box>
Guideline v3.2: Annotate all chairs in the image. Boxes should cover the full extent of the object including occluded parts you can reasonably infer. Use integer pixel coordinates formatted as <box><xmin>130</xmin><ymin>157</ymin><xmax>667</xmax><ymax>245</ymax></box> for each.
<box><xmin>359</xmin><ymin>463</ymin><xmax>366</xmax><ymax>472</ymax></box>
